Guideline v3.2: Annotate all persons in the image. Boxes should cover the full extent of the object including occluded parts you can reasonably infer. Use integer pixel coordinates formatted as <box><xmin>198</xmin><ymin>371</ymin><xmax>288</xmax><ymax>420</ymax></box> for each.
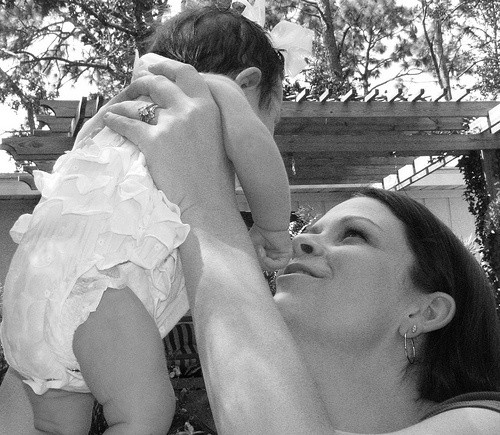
<box><xmin>1</xmin><ymin>5</ymin><xmax>295</xmax><ymax>435</ymax></box>
<box><xmin>102</xmin><ymin>51</ymin><xmax>500</xmax><ymax>434</ymax></box>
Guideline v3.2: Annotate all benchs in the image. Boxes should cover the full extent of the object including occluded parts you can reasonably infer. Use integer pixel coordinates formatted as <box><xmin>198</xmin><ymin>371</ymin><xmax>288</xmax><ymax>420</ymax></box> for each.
<box><xmin>159</xmin><ymin>316</ymin><xmax>203</xmax><ymax>379</ymax></box>
